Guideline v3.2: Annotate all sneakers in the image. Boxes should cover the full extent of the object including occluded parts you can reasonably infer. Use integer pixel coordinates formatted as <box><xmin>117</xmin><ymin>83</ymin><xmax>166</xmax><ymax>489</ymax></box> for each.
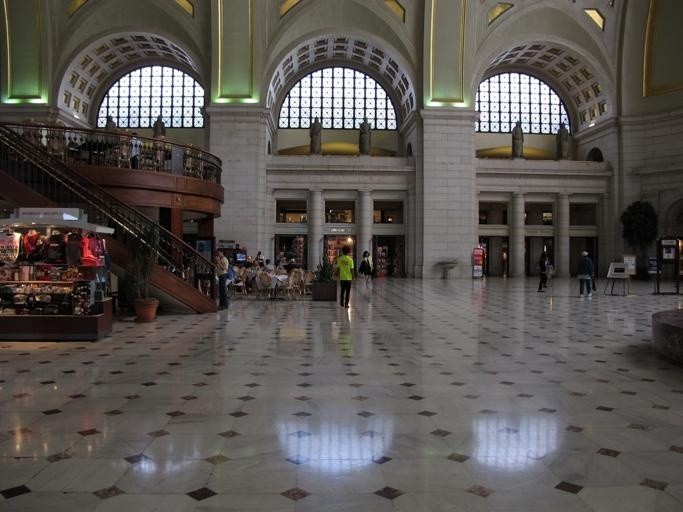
<box><xmin>536</xmin><ymin>285</ymin><xmax>593</xmax><ymax>298</ymax></box>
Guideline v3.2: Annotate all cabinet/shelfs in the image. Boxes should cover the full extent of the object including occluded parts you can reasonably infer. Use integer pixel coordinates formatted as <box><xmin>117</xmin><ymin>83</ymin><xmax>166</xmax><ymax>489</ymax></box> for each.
<box><xmin>0</xmin><ymin>217</ymin><xmax>115</xmax><ymax>342</ymax></box>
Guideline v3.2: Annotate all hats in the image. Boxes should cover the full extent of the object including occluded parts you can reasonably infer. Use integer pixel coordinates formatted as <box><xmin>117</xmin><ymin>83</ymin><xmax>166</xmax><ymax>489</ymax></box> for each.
<box><xmin>580</xmin><ymin>251</ymin><xmax>588</xmax><ymax>257</ymax></box>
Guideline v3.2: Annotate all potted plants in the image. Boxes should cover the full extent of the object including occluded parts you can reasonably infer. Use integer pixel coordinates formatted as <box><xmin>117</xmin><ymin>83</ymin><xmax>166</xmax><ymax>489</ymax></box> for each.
<box><xmin>127</xmin><ymin>219</ymin><xmax>161</xmax><ymax>323</ymax></box>
<box><xmin>310</xmin><ymin>249</ymin><xmax>338</xmax><ymax>301</ymax></box>
<box><xmin>620</xmin><ymin>200</ymin><xmax>659</xmax><ymax>281</ymax></box>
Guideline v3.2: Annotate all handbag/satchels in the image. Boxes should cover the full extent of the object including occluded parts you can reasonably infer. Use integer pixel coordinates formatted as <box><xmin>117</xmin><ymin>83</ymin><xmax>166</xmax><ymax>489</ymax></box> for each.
<box><xmin>0</xmin><ymin>226</ymin><xmax>107</xmax><ymax>319</ymax></box>
<box><xmin>592</xmin><ymin>278</ymin><xmax>597</xmax><ymax>291</ymax></box>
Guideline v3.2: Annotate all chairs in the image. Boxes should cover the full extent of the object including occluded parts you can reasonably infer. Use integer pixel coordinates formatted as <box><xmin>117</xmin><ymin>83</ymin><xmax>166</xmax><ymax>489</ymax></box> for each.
<box><xmin>231</xmin><ymin>265</ymin><xmax>316</xmax><ymax>302</ymax></box>
<box><xmin>603</xmin><ymin>262</ymin><xmax>630</xmax><ymax>297</ymax></box>
<box><xmin>20</xmin><ymin>117</ymin><xmax>205</xmax><ymax>177</ymax></box>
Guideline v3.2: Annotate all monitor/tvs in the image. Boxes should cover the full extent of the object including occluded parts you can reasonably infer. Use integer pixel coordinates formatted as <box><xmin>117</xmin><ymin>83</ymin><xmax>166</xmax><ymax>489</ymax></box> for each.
<box><xmin>236</xmin><ymin>253</ymin><xmax>247</xmax><ymax>262</ymax></box>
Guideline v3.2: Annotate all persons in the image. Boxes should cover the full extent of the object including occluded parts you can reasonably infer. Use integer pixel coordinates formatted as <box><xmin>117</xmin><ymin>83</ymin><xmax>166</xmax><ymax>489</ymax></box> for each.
<box><xmin>216</xmin><ymin>248</ymin><xmax>299</xmax><ymax>311</ymax></box>
<box><xmin>67</xmin><ymin>132</ymin><xmax>145</xmax><ymax>170</ymax></box>
<box><xmin>336</xmin><ymin>246</ymin><xmax>356</xmax><ymax>308</ymax></box>
<box><xmin>578</xmin><ymin>250</ymin><xmax>595</xmax><ymax>298</ymax></box>
<box><xmin>537</xmin><ymin>253</ymin><xmax>549</xmax><ymax>292</ymax></box>
<box><xmin>363</xmin><ymin>251</ymin><xmax>373</xmax><ymax>285</ymax></box>
